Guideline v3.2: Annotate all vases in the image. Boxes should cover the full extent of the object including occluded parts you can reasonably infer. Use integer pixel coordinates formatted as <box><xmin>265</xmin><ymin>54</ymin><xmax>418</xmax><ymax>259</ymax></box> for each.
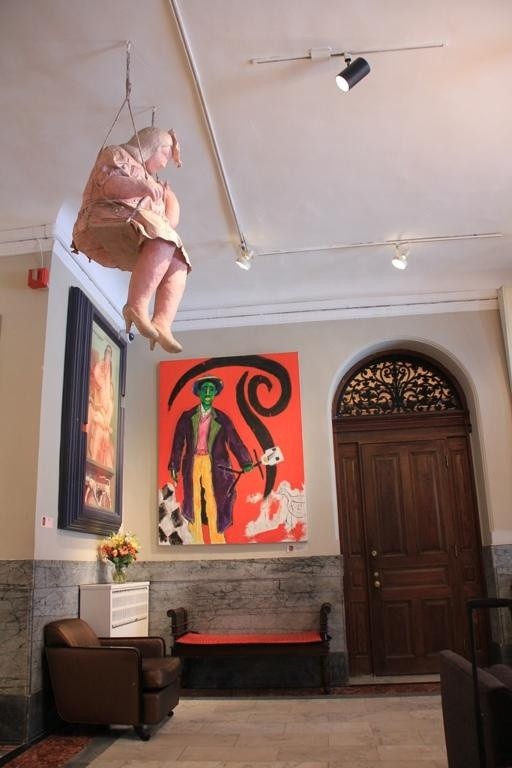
<box><xmin>111</xmin><ymin>565</ymin><xmax>129</xmax><ymax>583</ymax></box>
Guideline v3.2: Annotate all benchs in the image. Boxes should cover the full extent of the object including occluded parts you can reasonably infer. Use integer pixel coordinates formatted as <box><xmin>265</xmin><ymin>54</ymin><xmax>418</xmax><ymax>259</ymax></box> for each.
<box><xmin>167</xmin><ymin>601</ymin><xmax>332</xmax><ymax>696</ymax></box>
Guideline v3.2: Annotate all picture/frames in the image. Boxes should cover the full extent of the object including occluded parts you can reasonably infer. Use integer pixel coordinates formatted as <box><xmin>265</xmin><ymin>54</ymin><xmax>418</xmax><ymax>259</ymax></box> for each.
<box><xmin>56</xmin><ymin>285</ymin><xmax>129</xmax><ymax>537</ymax></box>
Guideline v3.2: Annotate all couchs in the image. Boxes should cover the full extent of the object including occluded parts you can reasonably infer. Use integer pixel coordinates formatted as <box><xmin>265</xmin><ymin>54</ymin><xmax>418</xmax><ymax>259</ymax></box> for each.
<box><xmin>42</xmin><ymin>618</ymin><xmax>184</xmax><ymax>741</ymax></box>
<box><xmin>438</xmin><ymin>646</ymin><xmax>511</xmax><ymax>767</ymax></box>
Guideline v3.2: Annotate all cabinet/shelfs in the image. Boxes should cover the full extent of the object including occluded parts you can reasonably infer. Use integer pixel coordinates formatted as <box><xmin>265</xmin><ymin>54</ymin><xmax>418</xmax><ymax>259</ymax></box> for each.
<box><xmin>78</xmin><ymin>580</ymin><xmax>152</xmax><ymax>637</ymax></box>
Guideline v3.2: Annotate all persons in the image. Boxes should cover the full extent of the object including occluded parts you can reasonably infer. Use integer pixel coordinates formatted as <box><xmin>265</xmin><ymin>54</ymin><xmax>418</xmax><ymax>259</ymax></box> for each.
<box><xmin>73</xmin><ymin>122</ymin><xmax>194</xmax><ymax>353</ymax></box>
<box><xmin>169</xmin><ymin>373</ymin><xmax>252</xmax><ymax>544</ymax></box>
<box><xmin>82</xmin><ymin>340</ymin><xmax>118</xmax><ymax>503</ymax></box>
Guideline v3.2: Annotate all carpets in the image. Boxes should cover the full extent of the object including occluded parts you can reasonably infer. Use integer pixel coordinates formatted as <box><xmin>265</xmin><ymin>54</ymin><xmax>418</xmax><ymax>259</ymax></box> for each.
<box><xmin>175</xmin><ymin>680</ymin><xmax>443</xmax><ymax>700</ymax></box>
<box><xmin>0</xmin><ymin>729</ymin><xmax>126</xmax><ymax>767</ymax></box>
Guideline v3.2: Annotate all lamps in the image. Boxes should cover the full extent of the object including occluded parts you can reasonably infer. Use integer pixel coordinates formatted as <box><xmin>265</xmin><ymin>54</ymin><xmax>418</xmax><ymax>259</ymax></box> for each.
<box><xmin>391</xmin><ymin>243</ymin><xmax>411</xmax><ymax>272</ymax></box>
<box><xmin>235</xmin><ymin>247</ymin><xmax>256</xmax><ymax>271</ymax></box>
<box><xmin>335</xmin><ymin>50</ymin><xmax>371</xmax><ymax>93</ymax></box>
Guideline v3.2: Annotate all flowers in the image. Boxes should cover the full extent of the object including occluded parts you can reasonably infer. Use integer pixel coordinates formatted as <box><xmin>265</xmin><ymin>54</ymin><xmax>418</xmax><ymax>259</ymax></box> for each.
<box><xmin>97</xmin><ymin>532</ymin><xmax>142</xmax><ymax>566</ymax></box>
